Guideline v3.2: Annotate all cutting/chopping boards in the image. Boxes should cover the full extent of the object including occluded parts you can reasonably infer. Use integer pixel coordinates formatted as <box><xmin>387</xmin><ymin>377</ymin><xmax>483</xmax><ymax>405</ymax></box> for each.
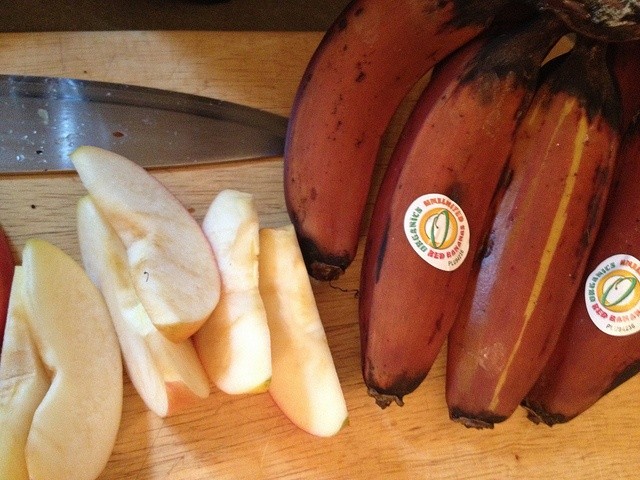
<box><xmin>2</xmin><ymin>31</ymin><xmax>639</xmax><ymax>480</ymax></box>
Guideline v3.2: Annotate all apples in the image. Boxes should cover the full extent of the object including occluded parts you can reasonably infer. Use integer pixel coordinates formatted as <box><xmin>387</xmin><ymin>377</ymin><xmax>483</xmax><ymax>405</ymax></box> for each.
<box><xmin>0</xmin><ymin>145</ymin><xmax>350</xmax><ymax>480</ymax></box>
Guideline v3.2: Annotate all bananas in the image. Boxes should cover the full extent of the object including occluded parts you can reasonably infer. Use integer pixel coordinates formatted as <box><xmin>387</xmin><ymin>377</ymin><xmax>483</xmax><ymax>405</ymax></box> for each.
<box><xmin>284</xmin><ymin>0</ymin><xmax>640</xmax><ymax>430</ymax></box>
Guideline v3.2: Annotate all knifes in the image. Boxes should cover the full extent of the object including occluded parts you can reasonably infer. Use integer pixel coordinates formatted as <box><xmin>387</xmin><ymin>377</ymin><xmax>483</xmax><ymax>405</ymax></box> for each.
<box><xmin>0</xmin><ymin>75</ymin><xmax>291</xmax><ymax>177</ymax></box>
